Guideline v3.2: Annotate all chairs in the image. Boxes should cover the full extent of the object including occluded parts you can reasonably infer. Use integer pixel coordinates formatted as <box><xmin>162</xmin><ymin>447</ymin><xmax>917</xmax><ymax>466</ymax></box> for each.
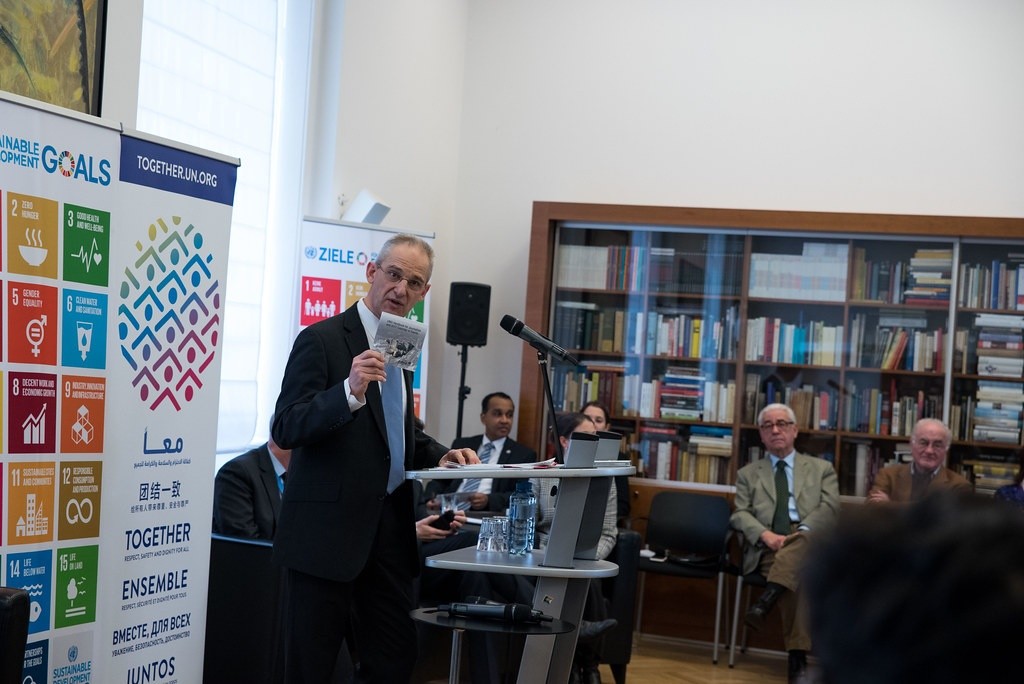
<box><xmin>0</xmin><ymin>587</ymin><xmax>30</xmax><ymax>684</ymax></box>
<box><xmin>727</xmin><ymin>551</ymin><xmax>769</xmax><ymax>669</ymax></box>
<box><xmin>632</xmin><ymin>488</ymin><xmax>735</xmax><ymax>666</ymax></box>
<box><xmin>203</xmin><ymin>532</ymin><xmax>355</xmax><ymax>684</ymax></box>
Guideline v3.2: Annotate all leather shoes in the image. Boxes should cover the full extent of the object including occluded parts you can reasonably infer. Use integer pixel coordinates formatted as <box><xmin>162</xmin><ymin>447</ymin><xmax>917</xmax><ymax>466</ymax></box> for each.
<box><xmin>579</xmin><ymin>619</ymin><xmax>618</xmax><ymax>644</ymax></box>
<box><xmin>789</xmin><ymin>663</ymin><xmax>808</xmax><ymax>684</ymax></box>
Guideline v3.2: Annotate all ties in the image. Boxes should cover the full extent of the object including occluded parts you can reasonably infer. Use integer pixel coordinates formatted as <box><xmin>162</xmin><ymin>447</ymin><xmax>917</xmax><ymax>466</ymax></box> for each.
<box><xmin>454</xmin><ymin>442</ymin><xmax>496</xmax><ymax>511</ymax></box>
<box><xmin>380</xmin><ymin>364</ymin><xmax>405</xmax><ymax>495</ymax></box>
<box><xmin>772</xmin><ymin>460</ymin><xmax>791</xmax><ymax>535</ymax></box>
<box><xmin>279</xmin><ymin>472</ymin><xmax>288</xmax><ymax>499</ymax></box>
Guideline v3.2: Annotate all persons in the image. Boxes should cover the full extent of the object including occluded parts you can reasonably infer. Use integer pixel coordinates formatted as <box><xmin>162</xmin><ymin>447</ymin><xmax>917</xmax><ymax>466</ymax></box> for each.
<box><xmin>799</xmin><ymin>418</ymin><xmax>1024</xmax><ymax>684</ymax></box>
<box><xmin>729</xmin><ymin>402</ymin><xmax>841</xmax><ymax>684</ymax></box>
<box><xmin>271</xmin><ymin>235</ymin><xmax>481</xmax><ymax>684</ymax></box>
<box><xmin>414</xmin><ymin>392</ymin><xmax>633</xmax><ymax>684</ymax></box>
<box><xmin>214</xmin><ymin>416</ymin><xmax>355</xmax><ymax>684</ymax></box>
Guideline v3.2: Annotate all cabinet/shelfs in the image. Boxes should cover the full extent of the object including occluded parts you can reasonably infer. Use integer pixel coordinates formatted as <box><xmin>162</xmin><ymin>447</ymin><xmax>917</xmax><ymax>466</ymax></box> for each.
<box><xmin>517</xmin><ymin>200</ymin><xmax>1024</xmax><ymax>661</ymax></box>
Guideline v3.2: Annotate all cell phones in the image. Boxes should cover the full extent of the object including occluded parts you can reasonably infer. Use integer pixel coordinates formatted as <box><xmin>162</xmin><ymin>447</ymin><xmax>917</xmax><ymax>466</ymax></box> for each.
<box><xmin>426</xmin><ymin>510</ymin><xmax>457</xmax><ymax>531</ymax></box>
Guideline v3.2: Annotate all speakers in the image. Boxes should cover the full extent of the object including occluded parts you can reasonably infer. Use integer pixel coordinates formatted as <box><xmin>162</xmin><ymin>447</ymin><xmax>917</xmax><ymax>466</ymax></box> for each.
<box><xmin>445</xmin><ymin>279</ymin><xmax>491</xmax><ymax>348</ymax></box>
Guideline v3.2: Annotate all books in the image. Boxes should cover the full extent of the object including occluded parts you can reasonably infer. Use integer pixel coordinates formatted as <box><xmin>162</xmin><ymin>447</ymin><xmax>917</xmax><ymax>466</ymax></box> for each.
<box><xmin>546</xmin><ymin>244</ymin><xmax>1023</xmax><ymax>493</ymax></box>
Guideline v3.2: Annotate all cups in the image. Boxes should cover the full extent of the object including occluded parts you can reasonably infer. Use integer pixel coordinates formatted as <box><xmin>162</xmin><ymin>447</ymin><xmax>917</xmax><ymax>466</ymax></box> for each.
<box><xmin>441</xmin><ymin>494</ymin><xmax>459</xmax><ymax>515</ymax></box>
<box><xmin>493</xmin><ymin>516</ymin><xmax>509</xmax><ymax>550</ymax></box>
<box><xmin>476</xmin><ymin>517</ymin><xmax>505</xmax><ymax>552</ymax></box>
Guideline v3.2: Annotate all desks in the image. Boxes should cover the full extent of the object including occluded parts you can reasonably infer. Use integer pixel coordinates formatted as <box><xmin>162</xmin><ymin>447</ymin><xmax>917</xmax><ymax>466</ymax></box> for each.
<box><xmin>409</xmin><ymin>606</ymin><xmax>576</xmax><ymax>684</ymax></box>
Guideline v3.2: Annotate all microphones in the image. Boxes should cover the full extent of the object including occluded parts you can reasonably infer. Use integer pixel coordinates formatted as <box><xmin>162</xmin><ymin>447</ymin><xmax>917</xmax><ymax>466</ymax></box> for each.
<box><xmin>439</xmin><ymin>594</ymin><xmax>553</xmax><ymax>625</ymax></box>
<box><xmin>499</xmin><ymin>312</ymin><xmax>588</xmax><ymax>372</ymax></box>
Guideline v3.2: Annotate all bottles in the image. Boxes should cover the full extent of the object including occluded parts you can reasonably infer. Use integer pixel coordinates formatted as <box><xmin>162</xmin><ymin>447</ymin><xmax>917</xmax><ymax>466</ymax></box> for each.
<box><xmin>526</xmin><ymin>482</ymin><xmax>536</xmax><ymax>552</ymax></box>
<box><xmin>507</xmin><ymin>483</ymin><xmax>531</xmax><ymax>553</ymax></box>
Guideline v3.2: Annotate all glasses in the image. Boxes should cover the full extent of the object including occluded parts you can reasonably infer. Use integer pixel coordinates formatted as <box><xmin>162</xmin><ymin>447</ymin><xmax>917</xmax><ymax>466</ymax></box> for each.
<box><xmin>375</xmin><ymin>261</ymin><xmax>427</xmax><ymax>292</ymax></box>
<box><xmin>759</xmin><ymin>420</ymin><xmax>794</xmax><ymax>431</ymax></box>
